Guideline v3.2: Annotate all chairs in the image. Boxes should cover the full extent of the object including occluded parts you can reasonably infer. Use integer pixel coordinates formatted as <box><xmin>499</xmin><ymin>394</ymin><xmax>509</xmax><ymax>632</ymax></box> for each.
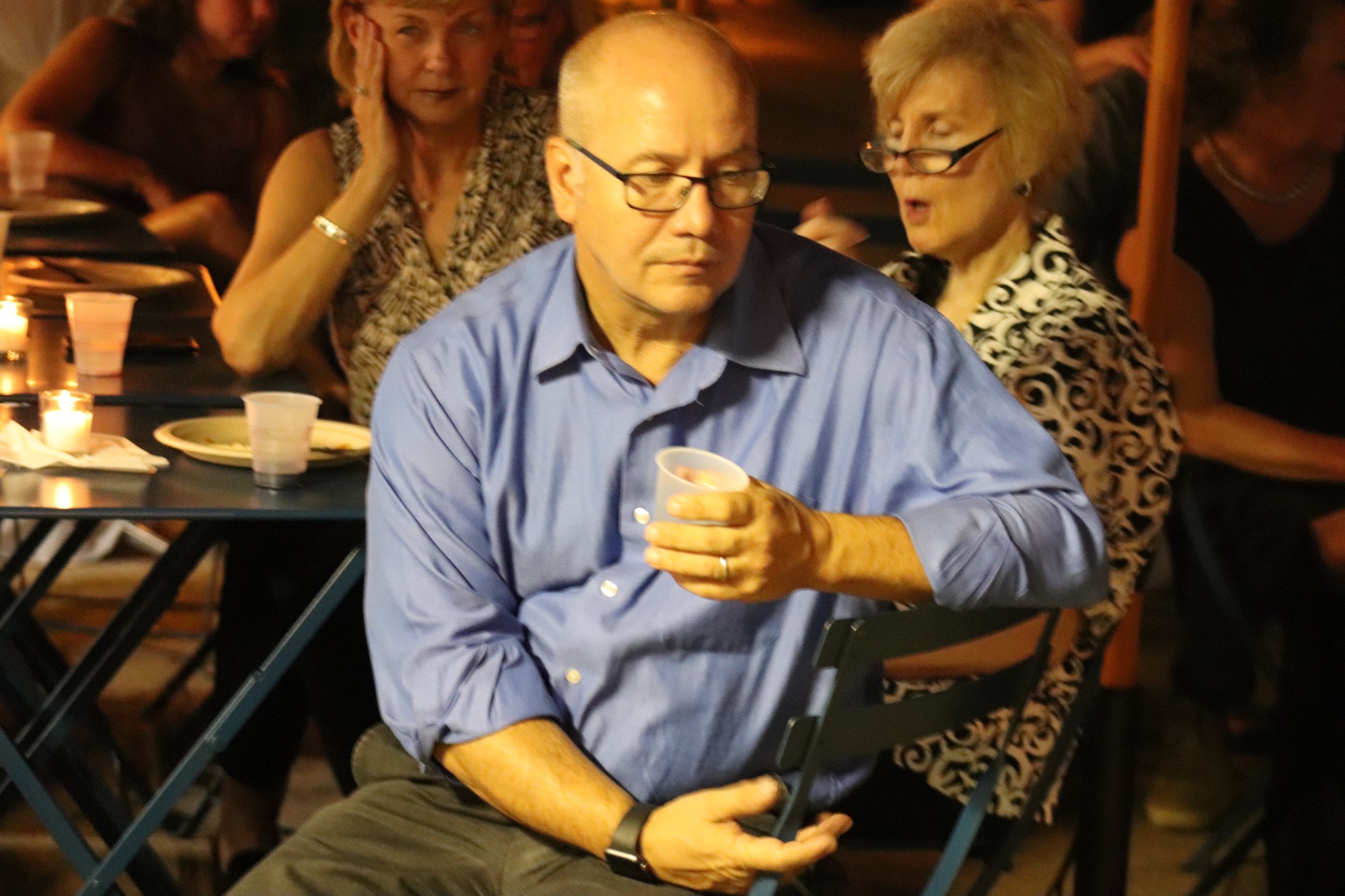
<box><xmin>746</xmin><ymin>601</ymin><xmax>1060</xmax><ymax>896</ymax></box>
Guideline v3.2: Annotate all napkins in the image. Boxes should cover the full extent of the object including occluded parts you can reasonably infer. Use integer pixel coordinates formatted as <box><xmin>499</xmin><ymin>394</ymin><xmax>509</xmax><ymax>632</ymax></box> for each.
<box><xmin>0</xmin><ymin>420</ymin><xmax>170</xmax><ymax>475</ymax></box>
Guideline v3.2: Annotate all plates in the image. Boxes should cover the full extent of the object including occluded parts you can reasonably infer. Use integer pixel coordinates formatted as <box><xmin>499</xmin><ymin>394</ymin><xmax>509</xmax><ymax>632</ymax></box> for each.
<box><xmin>0</xmin><ymin>193</ymin><xmax>110</xmax><ymax>226</ymax></box>
<box><xmin>6</xmin><ymin>258</ymin><xmax>194</xmax><ymax>299</ymax></box>
<box><xmin>153</xmin><ymin>414</ymin><xmax>370</xmax><ymax>468</ymax></box>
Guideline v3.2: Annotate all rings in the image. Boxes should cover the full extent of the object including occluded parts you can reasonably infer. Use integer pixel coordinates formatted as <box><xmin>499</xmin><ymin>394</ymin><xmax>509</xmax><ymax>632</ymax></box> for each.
<box><xmin>718</xmin><ymin>555</ymin><xmax>729</xmax><ymax>583</ymax></box>
<box><xmin>351</xmin><ymin>86</ymin><xmax>367</xmax><ymax>97</ymax></box>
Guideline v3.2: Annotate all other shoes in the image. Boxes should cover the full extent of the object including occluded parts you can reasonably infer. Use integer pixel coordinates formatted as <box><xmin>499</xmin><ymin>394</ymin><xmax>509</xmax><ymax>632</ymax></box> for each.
<box><xmin>210</xmin><ymin>824</ymin><xmax>282</xmax><ymax>896</ymax></box>
<box><xmin>1143</xmin><ymin>715</ymin><xmax>1235</xmax><ymax>827</ymax></box>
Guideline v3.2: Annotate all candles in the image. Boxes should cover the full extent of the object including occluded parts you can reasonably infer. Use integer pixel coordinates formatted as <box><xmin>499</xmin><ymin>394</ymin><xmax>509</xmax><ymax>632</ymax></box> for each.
<box><xmin>0</xmin><ymin>306</ymin><xmax>29</xmax><ymax>353</ymax></box>
<box><xmin>45</xmin><ymin>390</ymin><xmax>93</xmax><ymax>452</ymax></box>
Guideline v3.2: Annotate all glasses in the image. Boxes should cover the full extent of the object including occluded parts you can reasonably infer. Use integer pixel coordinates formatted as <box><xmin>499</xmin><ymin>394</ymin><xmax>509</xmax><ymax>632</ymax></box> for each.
<box><xmin>855</xmin><ymin>126</ymin><xmax>1005</xmax><ymax>175</ymax></box>
<box><xmin>565</xmin><ymin>137</ymin><xmax>771</xmax><ymax>214</ymax></box>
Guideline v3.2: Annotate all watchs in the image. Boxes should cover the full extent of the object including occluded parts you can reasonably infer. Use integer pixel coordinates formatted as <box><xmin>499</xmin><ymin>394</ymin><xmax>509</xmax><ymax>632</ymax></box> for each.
<box><xmin>605</xmin><ymin>802</ymin><xmax>660</xmax><ymax>884</ymax></box>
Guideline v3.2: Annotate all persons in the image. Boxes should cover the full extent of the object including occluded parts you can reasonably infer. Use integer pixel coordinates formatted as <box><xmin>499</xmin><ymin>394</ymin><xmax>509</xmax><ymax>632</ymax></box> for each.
<box><xmin>0</xmin><ymin>0</ymin><xmax>1345</xmax><ymax>896</ymax></box>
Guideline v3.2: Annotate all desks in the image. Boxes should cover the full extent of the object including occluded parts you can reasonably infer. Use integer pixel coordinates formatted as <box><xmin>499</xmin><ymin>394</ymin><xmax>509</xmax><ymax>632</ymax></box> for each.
<box><xmin>0</xmin><ymin>184</ymin><xmax>365</xmax><ymax>896</ymax></box>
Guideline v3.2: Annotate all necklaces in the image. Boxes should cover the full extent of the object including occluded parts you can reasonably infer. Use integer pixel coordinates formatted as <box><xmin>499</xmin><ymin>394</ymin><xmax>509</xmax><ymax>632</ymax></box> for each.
<box><xmin>1203</xmin><ymin>129</ymin><xmax>1317</xmax><ymax>204</ymax></box>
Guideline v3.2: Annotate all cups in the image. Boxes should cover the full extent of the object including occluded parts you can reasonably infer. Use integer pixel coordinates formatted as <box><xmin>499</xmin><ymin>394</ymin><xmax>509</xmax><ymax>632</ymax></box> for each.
<box><xmin>6</xmin><ymin>129</ymin><xmax>54</xmax><ymax>191</ymax></box>
<box><xmin>64</xmin><ymin>291</ymin><xmax>137</xmax><ymax>376</ymax></box>
<box><xmin>38</xmin><ymin>388</ymin><xmax>96</xmax><ymax>457</ymax></box>
<box><xmin>654</xmin><ymin>447</ymin><xmax>751</xmax><ymax>528</ymax></box>
<box><xmin>242</xmin><ymin>391</ymin><xmax>324</xmax><ymax>489</ymax></box>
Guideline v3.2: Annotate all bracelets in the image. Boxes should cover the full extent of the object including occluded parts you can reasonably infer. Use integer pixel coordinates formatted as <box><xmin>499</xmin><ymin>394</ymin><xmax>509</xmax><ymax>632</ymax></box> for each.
<box><xmin>311</xmin><ymin>213</ymin><xmax>365</xmax><ymax>253</ymax></box>
